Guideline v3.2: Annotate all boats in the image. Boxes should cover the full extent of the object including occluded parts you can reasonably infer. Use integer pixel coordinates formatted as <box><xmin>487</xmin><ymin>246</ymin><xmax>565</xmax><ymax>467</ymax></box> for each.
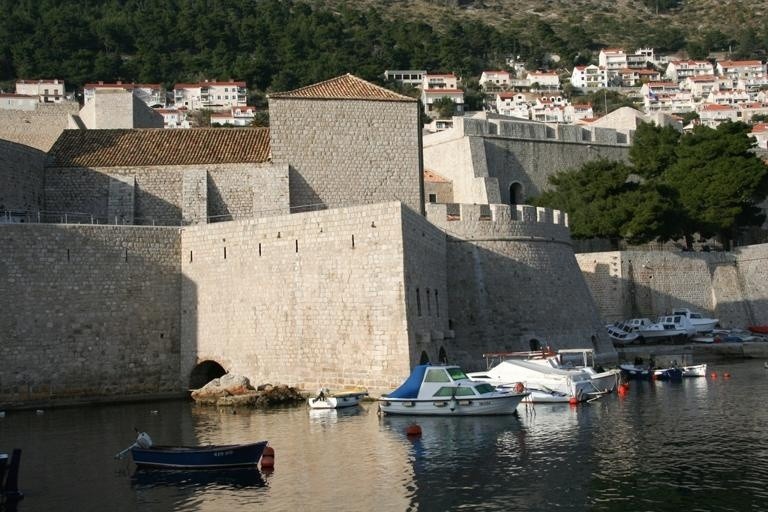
<box><xmin>113</xmin><ymin>430</ymin><xmax>276</xmax><ymax>473</ymax></box>
<box><xmin>604</xmin><ymin>305</ymin><xmax>767</xmax><ymax>344</ymax></box>
<box><xmin>308</xmin><ymin>387</ymin><xmax>369</xmax><ymax>410</ymax></box>
